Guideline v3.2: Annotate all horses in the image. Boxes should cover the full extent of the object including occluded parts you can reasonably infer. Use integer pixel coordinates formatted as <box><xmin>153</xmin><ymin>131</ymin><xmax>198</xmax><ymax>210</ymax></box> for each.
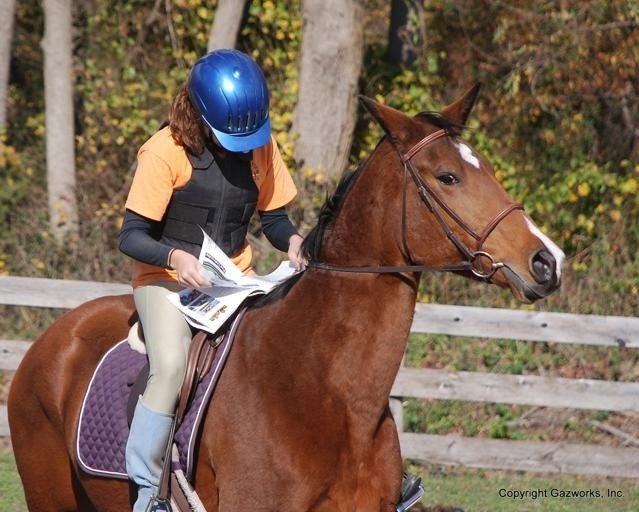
<box><xmin>7</xmin><ymin>80</ymin><xmax>567</xmax><ymax>512</ymax></box>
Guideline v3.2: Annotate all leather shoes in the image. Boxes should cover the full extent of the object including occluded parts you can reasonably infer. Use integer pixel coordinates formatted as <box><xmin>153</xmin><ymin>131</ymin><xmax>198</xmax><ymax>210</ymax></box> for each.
<box><xmin>400</xmin><ymin>473</ymin><xmax>422</xmax><ymax>501</ymax></box>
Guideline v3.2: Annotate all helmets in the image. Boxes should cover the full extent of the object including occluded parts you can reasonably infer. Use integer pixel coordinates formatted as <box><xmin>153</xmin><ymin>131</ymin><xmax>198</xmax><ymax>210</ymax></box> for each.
<box><xmin>187</xmin><ymin>48</ymin><xmax>271</xmax><ymax>153</ymax></box>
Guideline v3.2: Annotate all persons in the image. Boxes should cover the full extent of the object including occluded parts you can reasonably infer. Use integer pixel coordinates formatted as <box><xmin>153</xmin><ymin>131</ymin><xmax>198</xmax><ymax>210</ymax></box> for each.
<box><xmin>118</xmin><ymin>48</ymin><xmax>423</xmax><ymax>512</ymax></box>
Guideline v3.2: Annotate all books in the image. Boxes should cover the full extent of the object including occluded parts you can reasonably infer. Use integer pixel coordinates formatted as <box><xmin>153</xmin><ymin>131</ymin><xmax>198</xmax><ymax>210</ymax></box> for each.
<box><xmin>165</xmin><ymin>223</ymin><xmax>306</xmax><ymax>335</ymax></box>
